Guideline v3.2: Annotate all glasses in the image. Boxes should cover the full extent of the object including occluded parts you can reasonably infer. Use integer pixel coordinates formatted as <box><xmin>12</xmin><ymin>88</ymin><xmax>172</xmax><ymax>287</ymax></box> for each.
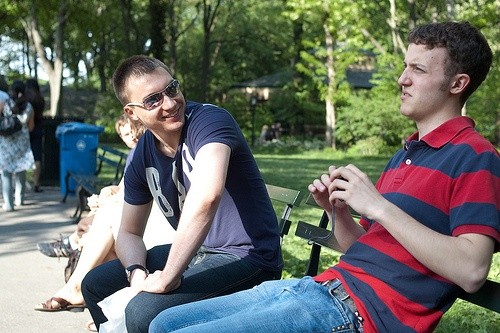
<box><xmin>125</xmin><ymin>79</ymin><xmax>181</xmax><ymax>110</ymax></box>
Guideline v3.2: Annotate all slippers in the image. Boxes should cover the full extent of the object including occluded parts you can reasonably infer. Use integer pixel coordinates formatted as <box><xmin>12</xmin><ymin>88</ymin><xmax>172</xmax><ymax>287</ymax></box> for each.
<box><xmin>33</xmin><ymin>296</ymin><xmax>86</xmax><ymax>312</ymax></box>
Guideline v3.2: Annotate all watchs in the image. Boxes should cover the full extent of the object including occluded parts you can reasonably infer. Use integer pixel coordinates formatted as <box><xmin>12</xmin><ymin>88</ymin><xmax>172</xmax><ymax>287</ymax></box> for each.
<box><xmin>126</xmin><ymin>264</ymin><xmax>149</xmax><ymax>282</ymax></box>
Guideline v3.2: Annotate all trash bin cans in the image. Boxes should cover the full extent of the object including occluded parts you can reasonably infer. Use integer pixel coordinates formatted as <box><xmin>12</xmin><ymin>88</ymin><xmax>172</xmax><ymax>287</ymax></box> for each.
<box><xmin>56</xmin><ymin>122</ymin><xmax>104</xmax><ymax>197</ymax></box>
<box><xmin>34</xmin><ymin>116</ymin><xmax>85</xmax><ymax>192</ymax></box>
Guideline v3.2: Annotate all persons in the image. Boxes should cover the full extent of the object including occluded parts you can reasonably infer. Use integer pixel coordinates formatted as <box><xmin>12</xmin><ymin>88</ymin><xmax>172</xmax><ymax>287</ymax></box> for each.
<box><xmin>37</xmin><ymin>111</ymin><xmax>137</xmax><ymax>283</ymax></box>
<box><xmin>0</xmin><ymin>75</ymin><xmax>46</xmax><ymax>210</ymax></box>
<box><xmin>34</xmin><ymin>115</ymin><xmax>176</xmax><ymax>330</ymax></box>
<box><xmin>81</xmin><ymin>56</ymin><xmax>283</xmax><ymax>333</ymax></box>
<box><xmin>149</xmin><ymin>21</ymin><xmax>500</xmax><ymax>332</ymax></box>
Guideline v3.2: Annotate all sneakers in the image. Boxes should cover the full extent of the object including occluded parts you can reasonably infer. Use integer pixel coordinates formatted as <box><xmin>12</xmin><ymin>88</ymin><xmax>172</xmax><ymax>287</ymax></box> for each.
<box><xmin>36</xmin><ymin>232</ymin><xmax>79</xmax><ymax>262</ymax></box>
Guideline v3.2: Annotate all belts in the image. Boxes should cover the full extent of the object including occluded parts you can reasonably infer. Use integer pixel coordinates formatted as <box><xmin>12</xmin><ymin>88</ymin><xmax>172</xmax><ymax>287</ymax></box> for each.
<box><xmin>321</xmin><ymin>278</ymin><xmax>365</xmax><ymax>324</ymax></box>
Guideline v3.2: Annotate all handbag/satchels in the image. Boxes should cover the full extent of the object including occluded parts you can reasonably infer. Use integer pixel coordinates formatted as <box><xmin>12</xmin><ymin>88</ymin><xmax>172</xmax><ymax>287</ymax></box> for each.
<box><xmin>0</xmin><ymin>99</ymin><xmax>22</xmax><ymax>137</ymax></box>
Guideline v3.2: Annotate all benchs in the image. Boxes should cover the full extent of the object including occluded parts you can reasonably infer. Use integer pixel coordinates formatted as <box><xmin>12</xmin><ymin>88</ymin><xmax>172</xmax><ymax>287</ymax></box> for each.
<box><xmin>62</xmin><ymin>147</ymin><xmax>500</xmax><ymax>315</ymax></box>
